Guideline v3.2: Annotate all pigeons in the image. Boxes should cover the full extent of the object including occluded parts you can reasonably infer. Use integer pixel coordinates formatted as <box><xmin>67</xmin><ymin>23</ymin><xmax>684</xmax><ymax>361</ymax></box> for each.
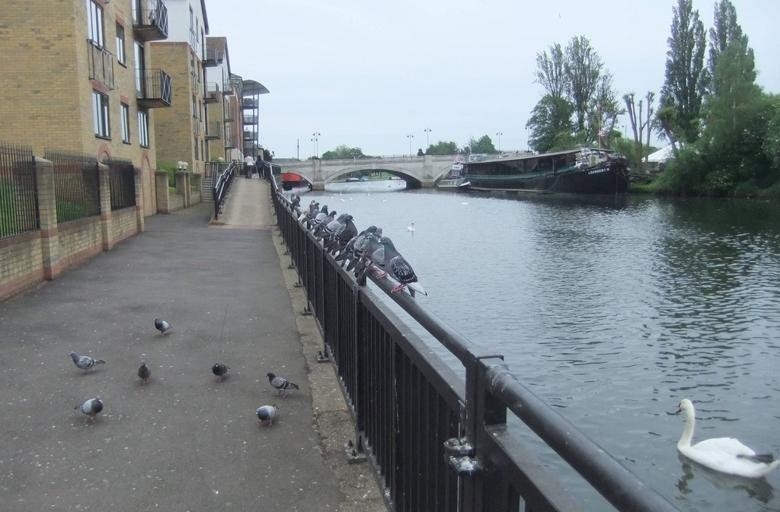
<box><xmin>265</xmin><ymin>372</ymin><xmax>299</xmax><ymax>396</ymax></box>
<box><xmin>154</xmin><ymin>317</ymin><xmax>174</xmax><ymax>337</ymax></box>
<box><xmin>256</xmin><ymin>404</ymin><xmax>280</xmax><ymax>426</ymax></box>
<box><xmin>68</xmin><ymin>352</ymin><xmax>106</xmax><ymax>374</ymax></box>
<box><xmin>74</xmin><ymin>395</ymin><xmax>104</xmax><ymax>423</ymax></box>
<box><xmin>138</xmin><ymin>362</ymin><xmax>152</xmax><ymax>384</ymax></box>
<box><xmin>212</xmin><ymin>362</ymin><xmax>231</xmax><ymax>382</ymax></box>
<box><xmin>288</xmin><ymin>193</ymin><xmax>385</xmax><ymax>286</ymax></box>
<box><xmin>377</xmin><ymin>237</ymin><xmax>427</xmax><ymax>297</ymax></box>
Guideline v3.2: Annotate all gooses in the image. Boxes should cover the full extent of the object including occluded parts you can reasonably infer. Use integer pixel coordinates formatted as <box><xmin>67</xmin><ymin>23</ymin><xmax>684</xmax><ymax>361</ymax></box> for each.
<box><xmin>407</xmin><ymin>222</ymin><xmax>415</xmax><ymax>231</ymax></box>
<box><xmin>675</xmin><ymin>398</ymin><xmax>780</xmax><ymax>478</ymax></box>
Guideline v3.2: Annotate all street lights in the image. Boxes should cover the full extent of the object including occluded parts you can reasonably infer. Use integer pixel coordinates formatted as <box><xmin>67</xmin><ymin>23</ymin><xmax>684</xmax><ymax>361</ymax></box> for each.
<box><xmin>424</xmin><ymin>129</ymin><xmax>431</xmax><ymax>147</ymax></box>
<box><xmin>525</xmin><ymin>125</ymin><xmax>532</xmax><ymax>151</ymax></box>
<box><xmin>407</xmin><ymin>134</ymin><xmax>414</xmax><ymax>156</ymax></box>
<box><xmin>310</xmin><ymin>130</ymin><xmax>322</xmax><ymax>160</ymax></box>
<box><xmin>496</xmin><ymin>132</ymin><xmax>503</xmax><ymax>154</ymax></box>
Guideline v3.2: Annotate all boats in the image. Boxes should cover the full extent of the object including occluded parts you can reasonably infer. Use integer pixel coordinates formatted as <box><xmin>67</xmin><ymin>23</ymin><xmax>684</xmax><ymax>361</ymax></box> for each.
<box><xmin>282</xmin><ymin>173</ymin><xmax>307</xmax><ymax>190</ymax></box>
<box><xmin>461</xmin><ymin>146</ymin><xmax>628</xmax><ymax>195</ymax></box>
<box><xmin>436</xmin><ymin>177</ymin><xmax>471</xmax><ymax>190</ymax></box>
<box><xmin>389</xmin><ymin>176</ymin><xmax>401</xmax><ymax>181</ymax></box>
<box><xmin>345</xmin><ymin>177</ymin><xmax>359</xmax><ymax>182</ymax></box>
<box><xmin>360</xmin><ymin>177</ymin><xmax>368</xmax><ymax>182</ymax></box>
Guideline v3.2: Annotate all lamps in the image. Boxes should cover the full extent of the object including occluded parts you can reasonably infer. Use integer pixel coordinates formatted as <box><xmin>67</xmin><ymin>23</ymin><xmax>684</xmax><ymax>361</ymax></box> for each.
<box><xmin>182</xmin><ymin>161</ymin><xmax>188</xmax><ymax>170</ymax></box>
<box><xmin>176</xmin><ymin>160</ymin><xmax>184</xmax><ymax>171</ymax></box>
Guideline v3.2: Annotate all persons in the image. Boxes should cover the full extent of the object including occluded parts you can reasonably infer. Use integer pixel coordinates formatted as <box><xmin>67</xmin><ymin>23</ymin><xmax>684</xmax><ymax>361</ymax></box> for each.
<box><xmin>244</xmin><ymin>153</ymin><xmax>255</xmax><ymax>178</ymax></box>
<box><xmin>255</xmin><ymin>155</ymin><xmax>265</xmax><ymax>178</ymax></box>
